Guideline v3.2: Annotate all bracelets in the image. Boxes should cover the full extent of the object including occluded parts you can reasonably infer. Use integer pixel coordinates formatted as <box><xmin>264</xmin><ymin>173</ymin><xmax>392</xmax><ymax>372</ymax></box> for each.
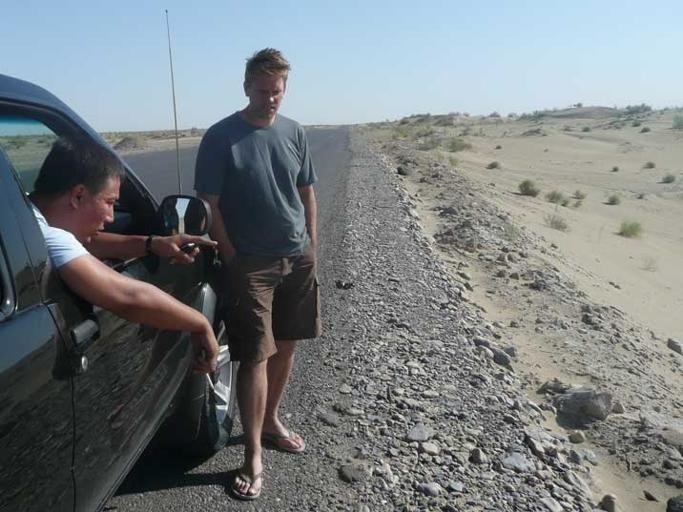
<box><xmin>147</xmin><ymin>235</ymin><xmax>157</xmax><ymax>255</ymax></box>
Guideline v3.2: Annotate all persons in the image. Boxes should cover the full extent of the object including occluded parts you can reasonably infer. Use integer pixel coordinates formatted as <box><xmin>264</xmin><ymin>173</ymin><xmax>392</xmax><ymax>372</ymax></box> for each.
<box><xmin>193</xmin><ymin>47</ymin><xmax>322</xmax><ymax>501</ymax></box>
<box><xmin>27</xmin><ymin>136</ymin><xmax>220</xmax><ymax>372</ymax></box>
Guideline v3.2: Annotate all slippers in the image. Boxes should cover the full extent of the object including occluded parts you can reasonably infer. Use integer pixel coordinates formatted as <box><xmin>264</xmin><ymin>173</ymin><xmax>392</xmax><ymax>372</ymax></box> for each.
<box><xmin>263</xmin><ymin>427</ymin><xmax>307</xmax><ymax>453</ymax></box>
<box><xmin>233</xmin><ymin>470</ymin><xmax>262</xmax><ymax>500</ymax></box>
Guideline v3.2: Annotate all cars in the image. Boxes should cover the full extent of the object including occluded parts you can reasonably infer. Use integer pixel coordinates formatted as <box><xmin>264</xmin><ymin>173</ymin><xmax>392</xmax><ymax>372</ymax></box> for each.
<box><xmin>0</xmin><ymin>74</ymin><xmax>238</xmax><ymax>512</ymax></box>
